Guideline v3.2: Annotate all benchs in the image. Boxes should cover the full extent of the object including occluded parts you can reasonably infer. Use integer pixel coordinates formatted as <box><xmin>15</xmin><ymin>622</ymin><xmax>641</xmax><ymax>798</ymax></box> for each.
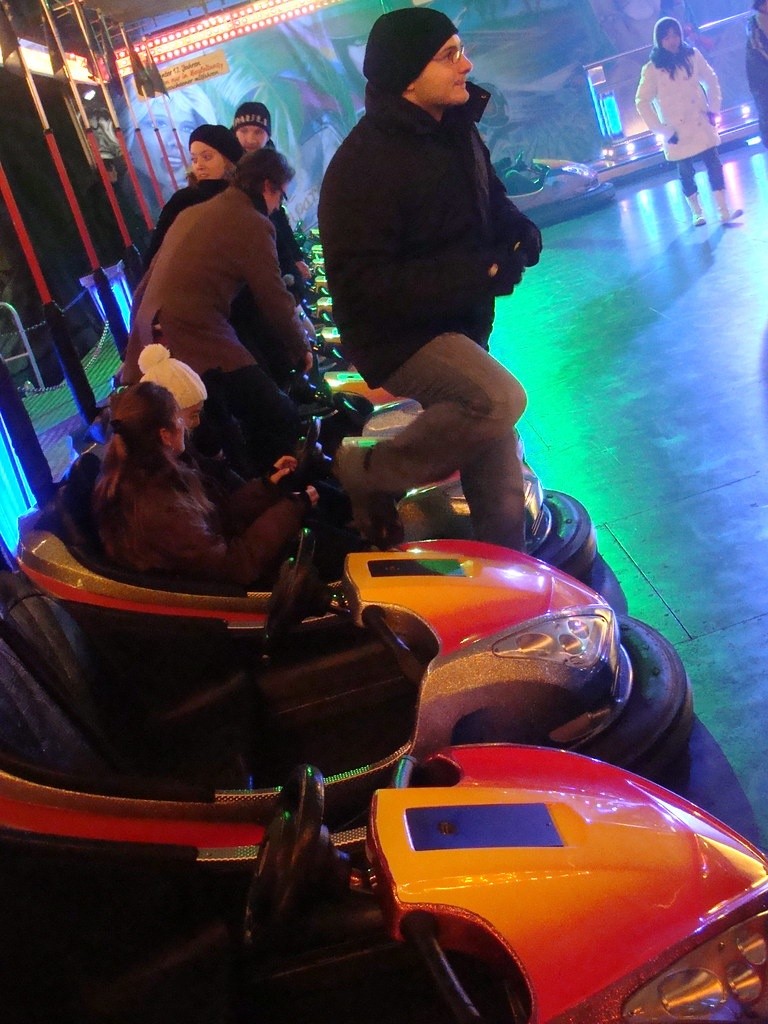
<box><xmin>1</xmin><ymin>829</ymin><xmax>235</xmax><ymax>1024</ymax></box>
<box><xmin>54</xmin><ymin>453</ymin><xmax>248</xmax><ymax>597</ymax></box>
<box><xmin>1</xmin><ymin>569</ymin><xmax>252</xmax><ymax>800</ymax></box>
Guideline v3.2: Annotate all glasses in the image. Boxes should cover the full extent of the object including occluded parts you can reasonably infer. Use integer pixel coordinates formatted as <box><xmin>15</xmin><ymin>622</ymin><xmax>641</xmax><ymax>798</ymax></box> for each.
<box><xmin>279</xmin><ymin>186</ymin><xmax>292</xmax><ymax>204</ymax></box>
<box><xmin>432</xmin><ymin>44</ymin><xmax>465</xmax><ymax>64</ymax></box>
<box><xmin>179</xmin><ymin>409</ymin><xmax>205</xmax><ymax>420</ymax></box>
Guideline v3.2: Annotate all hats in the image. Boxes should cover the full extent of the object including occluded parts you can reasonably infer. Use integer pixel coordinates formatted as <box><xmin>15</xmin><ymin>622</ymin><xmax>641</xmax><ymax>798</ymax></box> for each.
<box><xmin>138</xmin><ymin>342</ymin><xmax>208</xmax><ymax>408</ymax></box>
<box><xmin>189</xmin><ymin>124</ymin><xmax>243</xmax><ymax>164</ymax></box>
<box><xmin>363</xmin><ymin>8</ymin><xmax>458</xmax><ymax>95</ymax></box>
<box><xmin>234</xmin><ymin>102</ymin><xmax>272</xmax><ymax>136</ymax></box>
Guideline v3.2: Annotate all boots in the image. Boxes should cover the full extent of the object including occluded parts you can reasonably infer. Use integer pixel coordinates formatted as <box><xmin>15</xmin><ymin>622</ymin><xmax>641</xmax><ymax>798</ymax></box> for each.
<box><xmin>713</xmin><ymin>189</ymin><xmax>743</xmax><ymax>221</ymax></box>
<box><xmin>684</xmin><ymin>191</ymin><xmax>706</xmax><ymax>226</ymax></box>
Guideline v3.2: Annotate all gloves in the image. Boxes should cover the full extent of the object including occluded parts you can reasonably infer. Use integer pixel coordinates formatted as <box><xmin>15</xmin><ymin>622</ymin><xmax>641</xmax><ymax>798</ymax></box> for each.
<box><xmin>495</xmin><ymin>221</ymin><xmax>542</xmax><ymax>267</ymax></box>
<box><xmin>489</xmin><ymin>249</ymin><xmax>522</xmax><ymax>296</ymax></box>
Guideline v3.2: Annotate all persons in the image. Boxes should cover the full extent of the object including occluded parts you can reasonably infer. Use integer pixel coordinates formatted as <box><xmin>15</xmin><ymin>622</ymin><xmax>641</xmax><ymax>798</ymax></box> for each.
<box><xmin>479</xmin><ymin>134</ymin><xmax>539</xmax><ymax>196</ymax></box>
<box><xmin>95</xmin><ymin>100</ymin><xmax>387</xmax><ymax>594</ymax></box>
<box><xmin>635</xmin><ymin>16</ymin><xmax>743</xmax><ymax>227</ymax></box>
<box><xmin>745</xmin><ymin>1</ymin><xmax>767</xmax><ymax>147</ymax></box>
<box><xmin>316</xmin><ymin>7</ymin><xmax>544</xmax><ymax>557</ymax></box>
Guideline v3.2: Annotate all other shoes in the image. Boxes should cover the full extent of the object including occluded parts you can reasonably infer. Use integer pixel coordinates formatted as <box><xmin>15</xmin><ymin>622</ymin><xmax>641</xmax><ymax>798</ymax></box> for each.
<box><xmin>334</xmin><ymin>393</ymin><xmax>373</xmax><ymax>417</ymax></box>
<box><xmin>332</xmin><ymin>436</ymin><xmax>404</xmax><ymax>549</ymax></box>
